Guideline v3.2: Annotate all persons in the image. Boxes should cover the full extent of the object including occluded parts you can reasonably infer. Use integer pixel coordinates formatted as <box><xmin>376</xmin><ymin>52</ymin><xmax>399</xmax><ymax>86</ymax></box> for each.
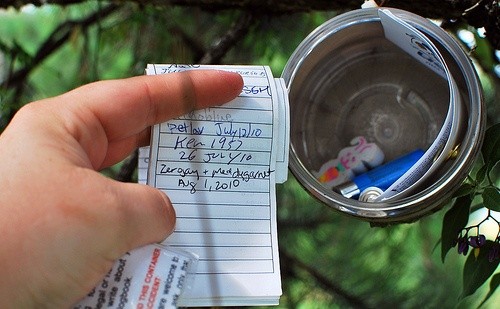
<box><xmin>0</xmin><ymin>68</ymin><xmax>245</xmax><ymax>309</ymax></box>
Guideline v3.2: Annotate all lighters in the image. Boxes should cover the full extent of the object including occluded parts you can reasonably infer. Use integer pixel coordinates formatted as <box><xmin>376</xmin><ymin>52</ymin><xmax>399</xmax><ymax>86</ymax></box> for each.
<box><xmin>333</xmin><ymin>149</ymin><xmax>435</xmax><ymax>202</ymax></box>
